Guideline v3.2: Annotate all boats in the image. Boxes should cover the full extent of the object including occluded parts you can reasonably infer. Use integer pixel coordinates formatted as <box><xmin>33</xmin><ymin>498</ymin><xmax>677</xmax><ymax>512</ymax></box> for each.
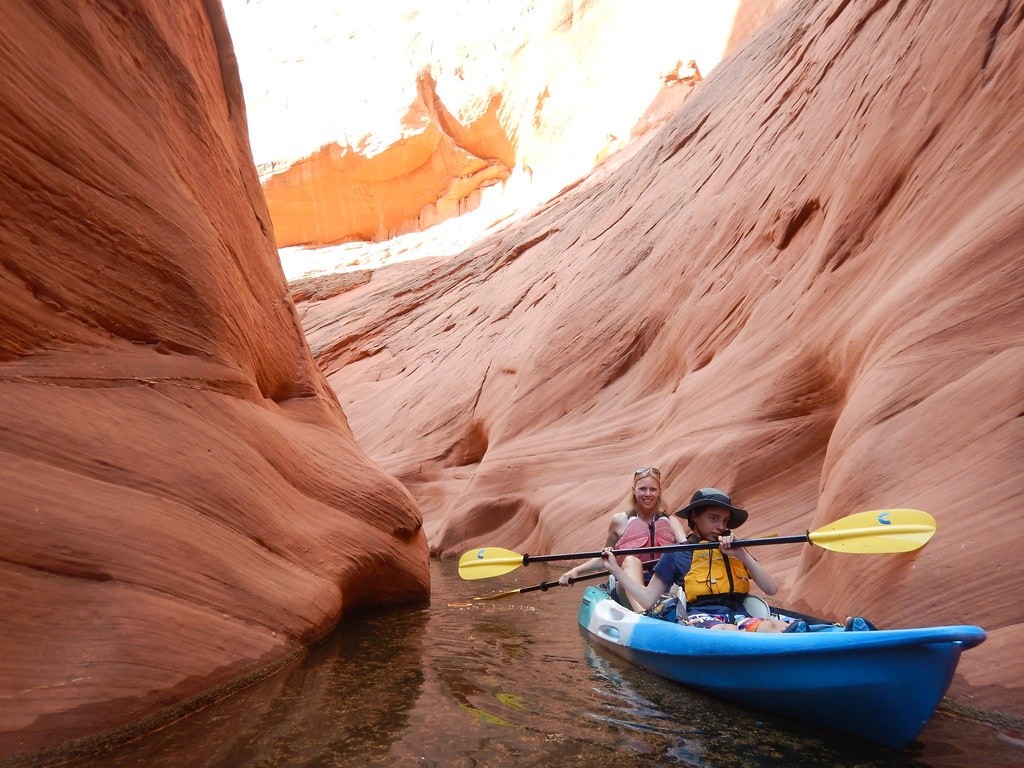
<box><xmin>580</xmin><ymin>573</ymin><xmax>986</xmax><ymax>755</ymax></box>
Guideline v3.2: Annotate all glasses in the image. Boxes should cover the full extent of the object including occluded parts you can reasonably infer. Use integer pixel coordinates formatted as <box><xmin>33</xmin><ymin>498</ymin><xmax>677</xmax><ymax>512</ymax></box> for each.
<box><xmin>634</xmin><ymin>467</ymin><xmax>660</xmax><ymax>479</ymax></box>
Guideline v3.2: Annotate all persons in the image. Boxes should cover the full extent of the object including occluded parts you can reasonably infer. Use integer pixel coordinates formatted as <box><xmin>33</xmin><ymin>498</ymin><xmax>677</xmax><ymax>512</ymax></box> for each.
<box><xmin>558</xmin><ymin>467</ymin><xmax>690</xmax><ymax>618</ymax></box>
<box><xmin>601</xmin><ymin>487</ymin><xmax>878</xmax><ymax>634</ymax></box>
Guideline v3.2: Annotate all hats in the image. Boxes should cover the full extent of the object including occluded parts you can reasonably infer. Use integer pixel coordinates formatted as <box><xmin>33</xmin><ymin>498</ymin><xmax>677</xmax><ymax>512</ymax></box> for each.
<box><xmin>674</xmin><ymin>488</ymin><xmax>748</xmax><ymax>530</ymax></box>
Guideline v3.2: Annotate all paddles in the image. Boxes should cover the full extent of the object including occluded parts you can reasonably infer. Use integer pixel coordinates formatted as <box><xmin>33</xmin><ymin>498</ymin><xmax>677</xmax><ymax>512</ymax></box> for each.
<box><xmin>456</xmin><ymin>506</ymin><xmax>939</xmax><ymax>584</ymax></box>
<box><xmin>472</xmin><ymin>531</ymin><xmax>780</xmax><ymax>601</ymax></box>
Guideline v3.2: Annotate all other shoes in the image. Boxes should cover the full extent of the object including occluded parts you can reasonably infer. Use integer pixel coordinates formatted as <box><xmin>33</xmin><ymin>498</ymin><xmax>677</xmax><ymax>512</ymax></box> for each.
<box><xmin>844</xmin><ymin>616</ymin><xmax>878</xmax><ymax>632</ymax></box>
<box><xmin>783</xmin><ymin>620</ymin><xmax>811</xmax><ymax>633</ymax></box>
